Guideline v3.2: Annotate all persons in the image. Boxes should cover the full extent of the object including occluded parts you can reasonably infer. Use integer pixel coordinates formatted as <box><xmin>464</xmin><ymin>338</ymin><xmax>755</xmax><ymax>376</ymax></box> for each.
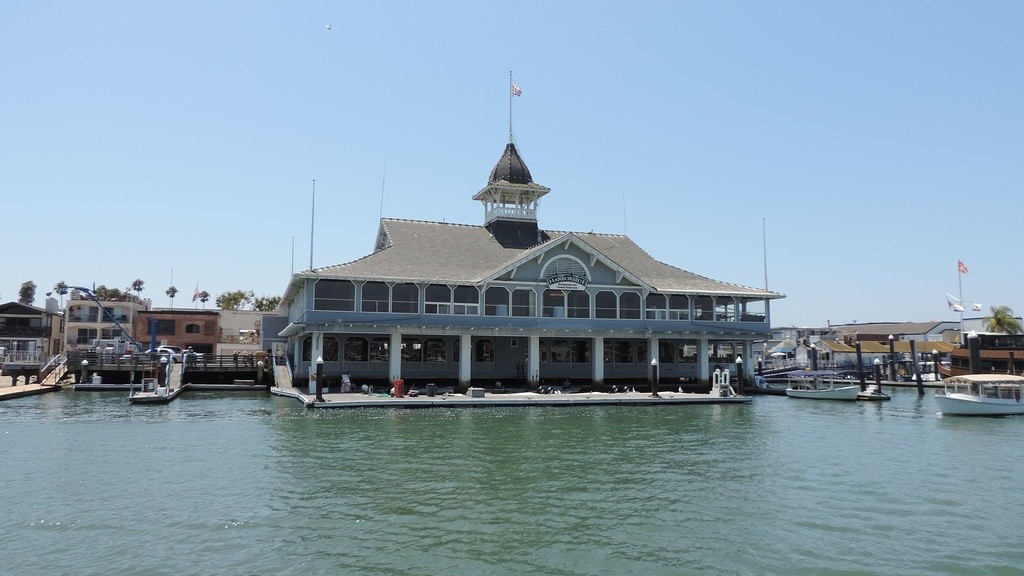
<box><xmin>182</xmin><ymin>346</ymin><xmax>197</xmax><ymax>365</ymax></box>
<box><xmin>232</xmin><ymin>351</ymin><xmax>254</xmax><ymax>364</ymax></box>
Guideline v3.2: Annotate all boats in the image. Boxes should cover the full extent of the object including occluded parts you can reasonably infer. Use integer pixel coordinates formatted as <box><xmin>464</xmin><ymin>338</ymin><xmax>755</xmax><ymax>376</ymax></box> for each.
<box><xmin>911</xmin><ymin>361</ymin><xmax>942</xmax><ymax>381</ymax></box>
<box><xmin>936</xmin><ymin>329</ymin><xmax>1024</xmax><ymax>376</ymax></box>
<box><xmin>934</xmin><ymin>373</ymin><xmax>1024</xmax><ymax>416</ymax></box>
<box><xmin>784</xmin><ymin>375</ymin><xmax>860</xmax><ymax>401</ymax></box>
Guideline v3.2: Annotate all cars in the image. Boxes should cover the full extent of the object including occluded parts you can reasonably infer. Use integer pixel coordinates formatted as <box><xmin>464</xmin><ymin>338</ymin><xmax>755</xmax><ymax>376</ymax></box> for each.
<box><xmin>121</xmin><ymin>345</ymin><xmax>203</xmax><ymax>364</ymax></box>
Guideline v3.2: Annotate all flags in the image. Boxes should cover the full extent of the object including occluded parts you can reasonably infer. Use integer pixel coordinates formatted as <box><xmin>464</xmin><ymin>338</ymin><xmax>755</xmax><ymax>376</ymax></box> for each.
<box><xmin>512</xmin><ymin>82</ymin><xmax>522</xmax><ymax>97</ymax></box>
<box><xmin>192</xmin><ymin>286</ymin><xmax>198</xmax><ymax>301</ymax></box>
<box><xmin>959</xmin><ymin>261</ymin><xmax>967</xmax><ymax>274</ymax></box>
